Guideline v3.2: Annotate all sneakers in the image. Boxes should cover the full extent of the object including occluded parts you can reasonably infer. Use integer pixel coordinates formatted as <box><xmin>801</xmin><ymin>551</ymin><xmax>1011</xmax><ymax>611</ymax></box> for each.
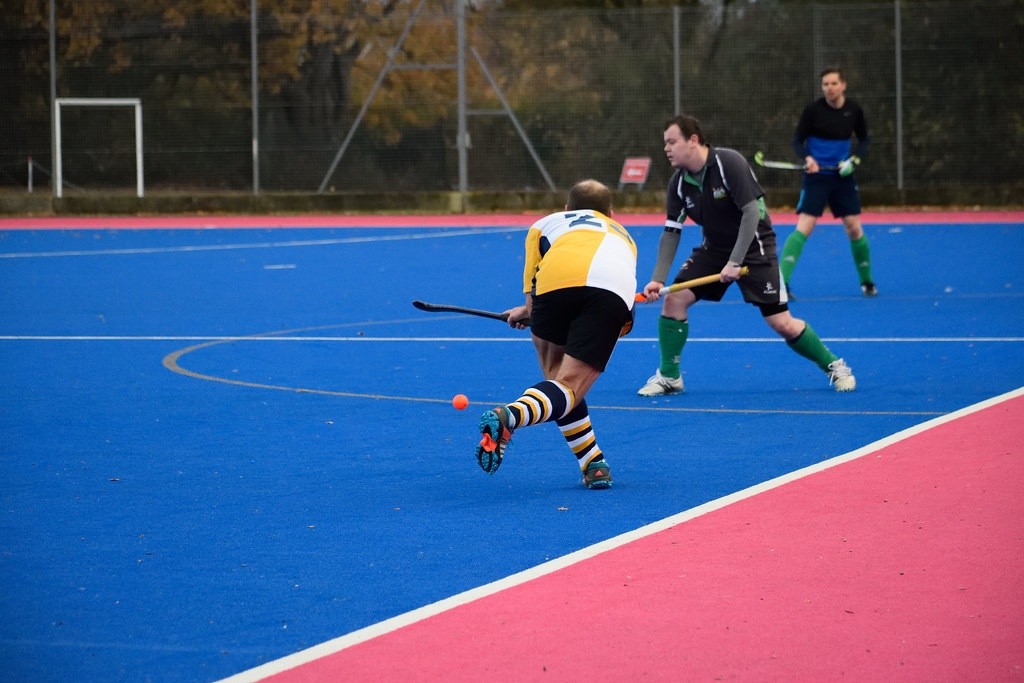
<box><xmin>825</xmin><ymin>358</ymin><xmax>855</xmax><ymax>391</ymax></box>
<box><xmin>637</xmin><ymin>368</ymin><xmax>684</xmax><ymax>398</ymax></box>
<box><xmin>474</xmin><ymin>407</ymin><xmax>515</xmax><ymax>475</ymax></box>
<box><xmin>581</xmin><ymin>458</ymin><xmax>613</xmax><ymax>489</ymax></box>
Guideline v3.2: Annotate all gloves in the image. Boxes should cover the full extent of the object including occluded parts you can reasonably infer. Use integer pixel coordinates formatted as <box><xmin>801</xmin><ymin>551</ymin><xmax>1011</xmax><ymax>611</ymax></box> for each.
<box><xmin>839</xmin><ymin>154</ymin><xmax>866</xmax><ymax>178</ymax></box>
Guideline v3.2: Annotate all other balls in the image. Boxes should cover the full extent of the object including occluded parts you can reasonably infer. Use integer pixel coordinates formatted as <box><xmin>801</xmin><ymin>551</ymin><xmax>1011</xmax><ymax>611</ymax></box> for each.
<box><xmin>452</xmin><ymin>393</ymin><xmax>469</xmax><ymax>410</ymax></box>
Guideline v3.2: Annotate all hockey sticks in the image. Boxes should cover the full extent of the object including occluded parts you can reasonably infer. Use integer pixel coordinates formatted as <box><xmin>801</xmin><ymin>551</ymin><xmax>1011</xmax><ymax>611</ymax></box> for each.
<box><xmin>411</xmin><ymin>299</ymin><xmax>533</xmax><ymax>328</ymax></box>
<box><xmin>635</xmin><ymin>265</ymin><xmax>751</xmax><ymax>305</ymax></box>
<box><xmin>754</xmin><ymin>150</ymin><xmax>840</xmax><ymax>172</ymax></box>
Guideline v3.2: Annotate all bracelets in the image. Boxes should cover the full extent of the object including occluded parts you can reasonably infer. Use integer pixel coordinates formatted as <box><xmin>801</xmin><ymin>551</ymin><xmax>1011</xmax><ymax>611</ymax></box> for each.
<box><xmin>727</xmin><ymin>262</ymin><xmax>739</xmax><ymax>267</ymax></box>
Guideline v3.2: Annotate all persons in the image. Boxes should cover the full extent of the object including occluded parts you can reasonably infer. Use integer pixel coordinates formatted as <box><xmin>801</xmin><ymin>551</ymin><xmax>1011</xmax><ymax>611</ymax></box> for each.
<box><xmin>475</xmin><ymin>180</ymin><xmax>638</xmax><ymax>486</ymax></box>
<box><xmin>638</xmin><ymin>115</ymin><xmax>856</xmax><ymax>396</ymax></box>
<box><xmin>780</xmin><ymin>68</ymin><xmax>877</xmax><ymax>300</ymax></box>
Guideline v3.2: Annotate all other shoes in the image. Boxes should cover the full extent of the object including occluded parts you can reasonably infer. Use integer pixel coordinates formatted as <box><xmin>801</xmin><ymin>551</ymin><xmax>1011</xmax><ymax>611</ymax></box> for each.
<box><xmin>860</xmin><ymin>282</ymin><xmax>878</xmax><ymax>296</ymax></box>
<box><xmin>785</xmin><ymin>284</ymin><xmax>795</xmax><ymax>301</ymax></box>
